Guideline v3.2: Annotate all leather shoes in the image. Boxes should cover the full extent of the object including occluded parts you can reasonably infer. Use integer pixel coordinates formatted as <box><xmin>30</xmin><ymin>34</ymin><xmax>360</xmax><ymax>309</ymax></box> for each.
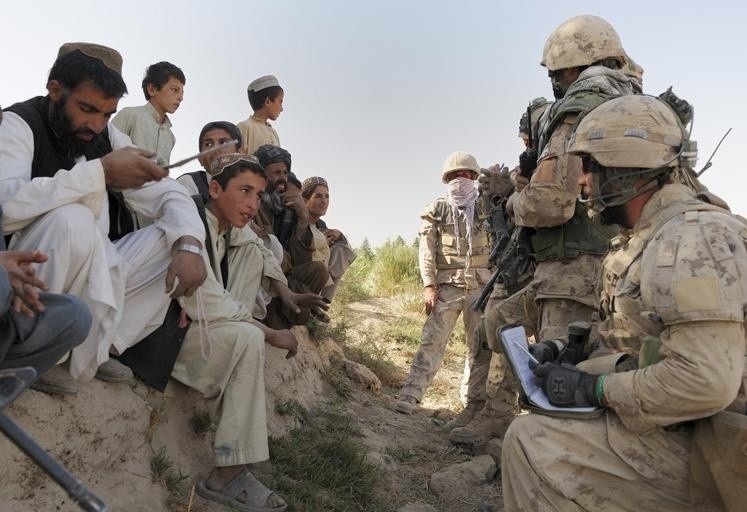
<box><xmin>95</xmin><ymin>358</ymin><xmax>134</xmax><ymax>382</ymax></box>
<box><xmin>29</xmin><ymin>366</ymin><xmax>78</xmax><ymax>396</ymax></box>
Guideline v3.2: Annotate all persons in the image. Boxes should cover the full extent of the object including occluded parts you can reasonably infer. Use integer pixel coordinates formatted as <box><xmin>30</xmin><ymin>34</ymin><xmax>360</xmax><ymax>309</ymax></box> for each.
<box><xmin>1</xmin><ymin>42</ymin><xmax>207</xmax><ymax>394</ymax></box>
<box><xmin>393</xmin><ymin>15</ymin><xmax>745</xmax><ymax>511</ymax></box>
<box><xmin>175</xmin><ymin>75</ymin><xmax>356</xmax><ymax>510</ymax></box>
<box><xmin>0</xmin><ymin>234</ymin><xmax>93</xmax><ymax>396</ymax></box>
<box><xmin>112</xmin><ymin>59</ymin><xmax>185</xmax><ymax>163</ymax></box>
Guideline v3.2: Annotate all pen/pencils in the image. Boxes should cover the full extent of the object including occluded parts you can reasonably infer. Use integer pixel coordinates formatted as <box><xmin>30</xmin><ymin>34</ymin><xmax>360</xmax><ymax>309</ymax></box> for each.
<box><xmin>477</xmin><ymin>162</ymin><xmax>505</xmax><ymax>199</ymax></box>
<box><xmin>179</xmin><ymin>294</ymin><xmax>187</xmax><ymax>329</ymax></box>
<box><xmin>512</xmin><ymin>339</ymin><xmax>543</xmax><ymax>367</ymax></box>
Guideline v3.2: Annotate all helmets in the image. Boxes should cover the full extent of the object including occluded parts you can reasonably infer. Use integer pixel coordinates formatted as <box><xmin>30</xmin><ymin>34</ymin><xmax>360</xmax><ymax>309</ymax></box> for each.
<box><xmin>540</xmin><ymin>15</ymin><xmax>626</xmax><ymax>72</ymax></box>
<box><xmin>518</xmin><ymin>101</ymin><xmax>555</xmax><ymax>140</ymax></box>
<box><xmin>565</xmin><ymin>93</ymin><xmax>684</xmax><ymax>169</ymax></box>
<box><xmin>442</xmin><ymin>152</ymin><xmax>480</xmax><ymax>184</ymax></box>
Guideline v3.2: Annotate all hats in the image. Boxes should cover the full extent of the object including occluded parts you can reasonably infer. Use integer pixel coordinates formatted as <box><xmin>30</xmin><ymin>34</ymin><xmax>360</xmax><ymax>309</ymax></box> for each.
<box><xmin>210</xmin><ymin>152</ymin><xmax>260</xmax><ymax>177</ymax></box>
<box><xmin>302</xmin><ymin>176</ymin><xmax>328</xmax><ymax>192</ymax></box>
<box><xmin>247</xmin><ymin>75</ymin><xmax>281</xmax><ymax>93</ymax></box>
<box><xmin>56</xmin><ymin>42</ymin><xmax>123</xmax><ymax>77</ymax></box>
<box><xmin>252</xmin><ymin>143</ymin><xmax>292</xmax><ymax>172</ymax></box>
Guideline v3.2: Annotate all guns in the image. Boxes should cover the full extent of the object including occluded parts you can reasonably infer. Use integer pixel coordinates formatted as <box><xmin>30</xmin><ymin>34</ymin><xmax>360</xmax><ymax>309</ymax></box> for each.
<box><xmin>474</xmin><ymin>225</ymin><xmax>526</xmax><ymax>312</ymax></box>
<box><xmin>505</xmin><ymin>106</ymin><xmax>536</xmax><ymax>282</ymax></box>
<box><xmin>0</xmin><ymin>367</ymin><xmax>108</xmax><ymax>512</ymax></box>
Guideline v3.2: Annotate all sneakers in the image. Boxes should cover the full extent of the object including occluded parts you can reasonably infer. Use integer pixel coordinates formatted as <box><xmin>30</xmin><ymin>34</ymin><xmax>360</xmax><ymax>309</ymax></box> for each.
<box><xmin>395</xmin><ymin>395</ymin><xmax>417</xmax><ymax>415</ymax></box>
<box><xmin>451</xmin><ymin>407</ymin><xmax>515</xmax><ymax>444</ymax></box>
<box><xmin>445</xmin><ymin>399</ymin><xmax>485</xmax><ymax>431</ymax></box>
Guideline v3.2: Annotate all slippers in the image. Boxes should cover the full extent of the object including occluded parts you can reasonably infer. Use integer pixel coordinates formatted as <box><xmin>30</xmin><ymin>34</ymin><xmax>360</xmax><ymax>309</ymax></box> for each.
<box><xmin>197</xmin><ymin>468</ymin><xmax>287</xmax><ymax>511</ymax></box>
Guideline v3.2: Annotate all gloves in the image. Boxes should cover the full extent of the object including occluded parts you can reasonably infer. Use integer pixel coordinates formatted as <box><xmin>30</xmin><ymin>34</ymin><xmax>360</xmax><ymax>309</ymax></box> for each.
<box><xmin>478</xmin><ymin>166</ymin><xmax>515</xmax><ymax>198</ymax></box>
<box><xmin>534</xmin><ymin>361</ymin><xmax>599</xmax><ymax>409</ymax></box>
<box><xmin>516</xmin><ymin>174</ymin><xmax>528</xmax><ymax>193</ymax></box>
<box><xmin>528</xmin><ymin>340</ymin><xmax>559</xmax><ymax>369</ymax></box>
